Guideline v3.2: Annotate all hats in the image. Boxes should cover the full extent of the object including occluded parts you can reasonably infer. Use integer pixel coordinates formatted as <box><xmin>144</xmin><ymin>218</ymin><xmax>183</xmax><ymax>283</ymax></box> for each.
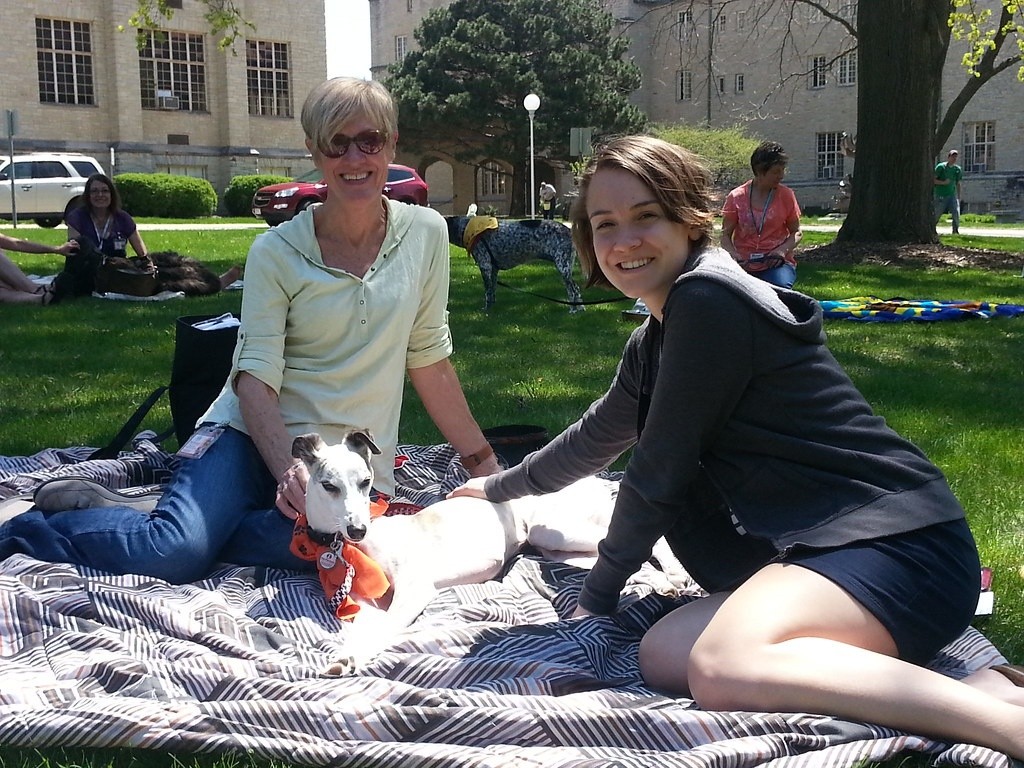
<box><xmin>948</xmin><ymin>150</ymin><xmax>959</xmax><ymax>156</ymax></box>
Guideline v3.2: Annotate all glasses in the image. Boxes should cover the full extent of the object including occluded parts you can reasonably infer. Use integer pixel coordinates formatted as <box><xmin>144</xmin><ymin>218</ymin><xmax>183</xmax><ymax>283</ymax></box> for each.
<box><xmin>90</xmin><ymin>186</ymin><xmax>110</xmax><ymax>195</ymax></box>
<box><xmin>317</xmin><ymin>129</ymin><xmax>390</xmax><ymax>159</ymax></box>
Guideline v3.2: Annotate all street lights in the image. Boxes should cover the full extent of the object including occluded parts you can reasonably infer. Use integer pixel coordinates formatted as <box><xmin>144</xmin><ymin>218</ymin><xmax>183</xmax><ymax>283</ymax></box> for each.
<box><xmin>524</xmin><ymin>91</ymin><xmax>540</xmax><ymax>219</ymax></box>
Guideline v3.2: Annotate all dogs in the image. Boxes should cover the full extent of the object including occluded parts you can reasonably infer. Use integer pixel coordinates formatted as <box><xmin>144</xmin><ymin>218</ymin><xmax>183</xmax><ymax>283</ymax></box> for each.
<box><xmin>444</xmin><ymin>215</ymin><xmax>586</xmax><ymax>313</ymax></box>
<box><xmin>292</xmin><ymin>429</ymin><xmax>690</xmax><ymax>674</ymax></box>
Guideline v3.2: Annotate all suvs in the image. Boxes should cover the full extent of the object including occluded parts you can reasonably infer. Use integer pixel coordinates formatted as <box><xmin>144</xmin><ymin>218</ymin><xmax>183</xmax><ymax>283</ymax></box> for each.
<box><xmin>0</xmin><ymin>154</ymin><xmax>106</xmax><ymax>228</ymax></box>
<box><xmin>251</xmin><ymin>163</ymin><xmax>430</xmax><ymax>226</ymax></box>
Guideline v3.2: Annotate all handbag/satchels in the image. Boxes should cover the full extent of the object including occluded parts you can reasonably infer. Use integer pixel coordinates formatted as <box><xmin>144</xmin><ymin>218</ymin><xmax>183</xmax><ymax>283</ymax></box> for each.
<box><xmin>543</xmin><ymin>201</ymin><xmax>550</xmax><ymax>210</ymax></box>
<box><xmin>169</xmin><ymin>313</ymin><xmax>241</xmax><ymax>447</ymax></box>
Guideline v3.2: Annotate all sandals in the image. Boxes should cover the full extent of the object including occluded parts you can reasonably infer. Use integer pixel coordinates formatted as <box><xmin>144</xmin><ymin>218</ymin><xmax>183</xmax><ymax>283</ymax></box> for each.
<box><xmin>33</xmin><ymin>282</ymin><xmax>53</xmax><ymax>294</ymax></box>
<box><xmin>42</xmin><ymin>282</ymin><xmax>57</xmax><ymax>306</ymax></box>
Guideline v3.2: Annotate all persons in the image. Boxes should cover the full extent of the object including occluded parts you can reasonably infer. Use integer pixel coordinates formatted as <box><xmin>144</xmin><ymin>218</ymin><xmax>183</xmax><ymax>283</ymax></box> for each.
<box><xmin>444</xmin><ymin>135</ymin><xmax>1023</xmax><ymax>758</ymax></box>
<box><xmin>0</xmin><ymin>76</ymin><xmax>503</xmax><ymax>585</ymax></box>
<box><xmin>0</xmin><ymin>172</ymin><xmax>238</xmax><ymax>306</ymax></box>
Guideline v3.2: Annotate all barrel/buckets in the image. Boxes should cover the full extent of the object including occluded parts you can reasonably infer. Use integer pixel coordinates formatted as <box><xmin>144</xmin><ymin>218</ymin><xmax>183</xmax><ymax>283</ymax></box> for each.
<box><xmin>483</xmin><ymin>425</ymin><xmax>549</xmax><ymax>468</ymax></box>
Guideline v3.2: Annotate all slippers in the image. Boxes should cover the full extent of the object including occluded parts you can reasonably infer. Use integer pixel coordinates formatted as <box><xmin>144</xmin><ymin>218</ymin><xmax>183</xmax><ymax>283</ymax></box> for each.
<box><xmin>989</xmin><ymin>663</ymin><xmax>1024</xmax><ymax>687</ymax></box>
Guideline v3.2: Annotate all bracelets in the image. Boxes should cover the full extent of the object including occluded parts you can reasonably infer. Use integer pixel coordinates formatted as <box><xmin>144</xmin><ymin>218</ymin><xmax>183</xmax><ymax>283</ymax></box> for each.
<box><xmin>461</xmin><ymin>441</ymin><xmax>493</xmax><ymax>470</ymax></box>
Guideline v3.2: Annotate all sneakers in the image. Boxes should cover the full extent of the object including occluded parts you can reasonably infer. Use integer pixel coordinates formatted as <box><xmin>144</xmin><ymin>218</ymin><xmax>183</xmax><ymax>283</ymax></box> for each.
<box><xmin>0</xmin><ymin>494</ymin><xmax>35</xmax><ymax>526</ymax></box>
<box><xmin>34</xmin><ymin>476</ymin><xmax>164</xmax><ymax>515</ymax></box>
